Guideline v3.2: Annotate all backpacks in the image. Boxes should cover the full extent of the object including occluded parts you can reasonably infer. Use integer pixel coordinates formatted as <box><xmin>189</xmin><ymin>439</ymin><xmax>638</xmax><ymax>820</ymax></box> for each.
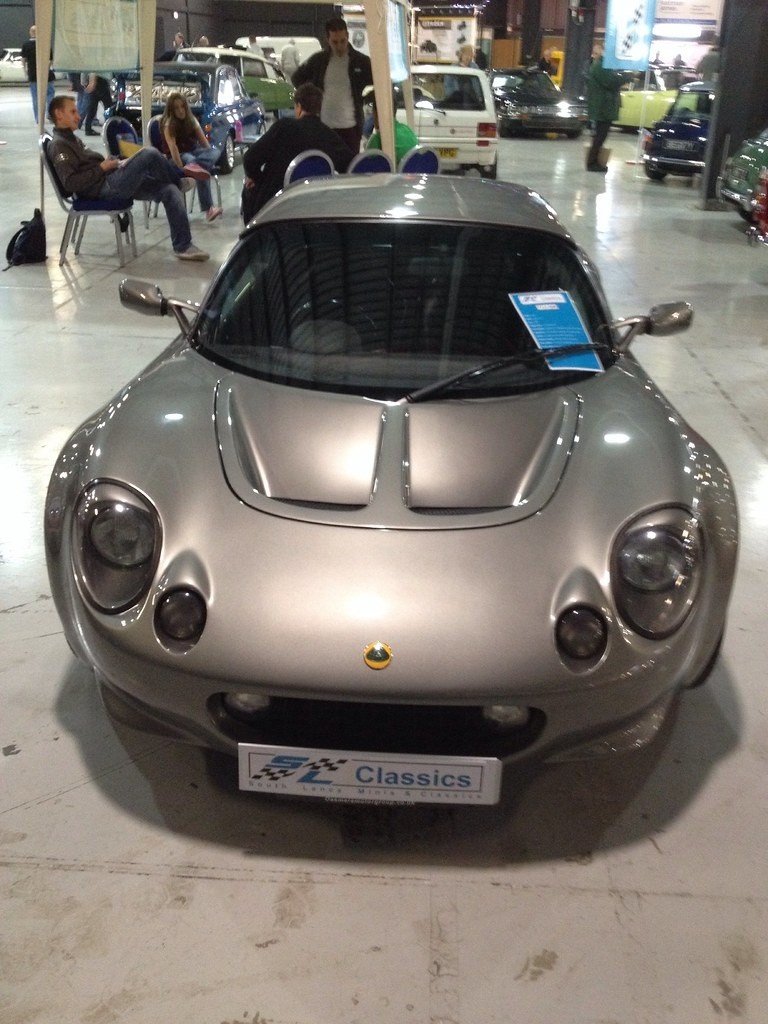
<box><xmin>6</xmin><ymin>209</ymin><xmax>46</xmax><ymax>266</ymax></box>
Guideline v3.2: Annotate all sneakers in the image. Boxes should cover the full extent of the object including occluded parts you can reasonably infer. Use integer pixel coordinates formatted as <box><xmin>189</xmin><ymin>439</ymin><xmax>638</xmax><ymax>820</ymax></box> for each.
<box><xmin>205</xmin><ymin>205</ymin><xmax>223</xmax><ymax>221</ymax></box>
<box><xmin>174</xmin><ymin>244</ymin><xmax>208</xmax><ymax>261</ymax></box>
<box><xmin>184</xmin><ymin>163</ymin><xmax>211</xmax><ymax>180</ymax></box>
<box><xmin>180</xmin><ymin>177</ymin><xmax>196</xmax><ymax>194</ymax></box>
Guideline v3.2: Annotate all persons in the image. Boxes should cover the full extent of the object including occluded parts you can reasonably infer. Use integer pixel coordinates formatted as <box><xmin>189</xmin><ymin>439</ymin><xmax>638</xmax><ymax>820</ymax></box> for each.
<box><xmin>281</xmin><ymin>39</ymin><xmax>300</xmax><ymax>79</ymax></box>
<box><xmin>585</xmin><ymin>43</ymin><xmax>641</xmax><ymax>172</ymax></box>
<box><xmin>21</xmin><ymin>25</ymin><xmax>56</xmax><ymax>125</ymax></box>
<box><xmin>159</xmin><ymin>92</ymin><xmax>224</xmax><ymax>223</ymax></box>
<box><xmin>44</xmin><ymin>95</ymin><xmax>211</xmax><ymax>264</ymax></box>
<box><xmin>175</xmin><ymin>32</ymin><xmax>191</xmax><ymax>48</ymax></box>
<box><xmin>292</xmin><ymin>18</ymin><xmax>376</xmax><ymax>159</ymax></box>
<box><xmin>694</xmin><ymin>35</ymin><xmax>723</xmax><ymax>84</ymax></box>
<box><xmin>455</xmin><ymin>34</ymin><xmax>488</xmax><ymax>70</ymax></box>
<box><xmin>672</xmin><ymin>54</ymin><xmax>689</xmax><ymax>68</ymax></box>
<box><xmin>67</xmin><ymin>70</ymin><xmax>118</xmax><ymax>136</ymax></box>
<box><xmin>653</xmin><ymin>51</ymin><xmax>665</xmax><ymax>65</ymax></box>
<box><xmin>538</xmin><ymin>49</ymin><xmax>553</xmax><ymax>79</ymax></box>
<box><xmin>241</xmin><ymin>82</ymin><xmax>355</xmax><ymax>225</ymax></box>
<box><xmin>245</xmin><ymin>35</ymin><xmax>266</xmax><ymax>59</ymax></box>
<box><xmin>365</xmin><ymin>96</ymin><xmax>418</xmax><ymax>171</ymax></box>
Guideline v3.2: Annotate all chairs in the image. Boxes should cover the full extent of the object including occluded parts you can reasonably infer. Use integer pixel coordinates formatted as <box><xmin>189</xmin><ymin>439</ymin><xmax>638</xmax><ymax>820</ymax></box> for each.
<box><xmin>37</xmin><ymin>114</ymin><xmax>222</xmax><ymax>268</ymax></box>
<box><xmin>440</xmin><ymin>89</ymin><xmax>479</xmax><ymax>108</ymax></box>
<box><xmin>397</xmin><ymin>88</ymin><xmax>423</xmax><ymax>108</ymax></box>
<box><xmin>397</xmin><ymin>145</ymin><xmax>441</xmax><ymax>177</ymax></box>
<box><xmin>349</xmin><ymin>149</ymin><xmax>393</xmax><ymax>174</ymax></box>
<box><xmin>283</xmin><ymin>149</ymin><xmax>334</xmax><ymax>190</ymax></box>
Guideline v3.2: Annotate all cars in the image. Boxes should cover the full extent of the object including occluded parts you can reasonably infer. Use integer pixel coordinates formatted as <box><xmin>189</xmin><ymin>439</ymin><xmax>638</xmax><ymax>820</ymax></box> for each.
<box><xmin>364</xmin><ymin>64</ymin><xmax>498</xmax><ymax>179</ymax></box>
<box><xmin>43</xmin><ymin>175</ymin><xmax>739</xmax><ymax>806</ymax></box>
<box><xmin>610</xmin><ymin>70</ymin><xmax>701</xmax><ymax>130</ymax></box>
<box><xmin>639</xmin><ymin>80</ymin><xmax>718</xmax><ymax>180</ymax></box>
<box><xmin>0</xmin><ymin>48</ymin><xmax>29</xmax><ymax>83</ymax></box>
<box><xmin>172</xmin><ymin>47</ymin><xmax>297</xmax><ymax>118</ymax></box>
<box><xmin>102</xmin><ymin>61</ymin><xmax>268</xmax><ymax>174</ymax></box>
<box><xmin>487</xmin><ymin>67</ymin><xmax>589</xmax><ymax>139</ymax></box>
<box><xmin>716</xmin><ymin>127</ymin><xmax>768</xmax><ymax>231</ymax></box>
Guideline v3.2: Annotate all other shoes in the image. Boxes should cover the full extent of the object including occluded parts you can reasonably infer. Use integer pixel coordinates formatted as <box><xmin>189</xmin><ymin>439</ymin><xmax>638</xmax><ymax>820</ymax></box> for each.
<box><xmin>86</xmin><ymin>130</ymin><xmax>100</xmax><ymax>135</ymax></box>
<box><xmin>590</xmin><ymin>165</ymin><xmax>608</xmax><ymax>172</ymax></box>
